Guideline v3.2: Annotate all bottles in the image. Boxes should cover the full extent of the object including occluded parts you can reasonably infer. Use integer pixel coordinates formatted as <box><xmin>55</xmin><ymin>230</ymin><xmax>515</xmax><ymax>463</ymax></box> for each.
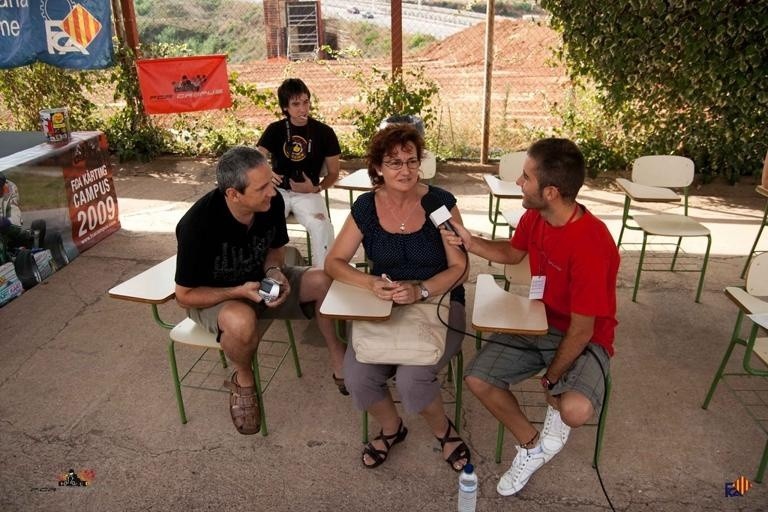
<box><xmin>456</xmin><ymin>463</ymin><xmax>479</xmax><ymax>511</ymax></box>
<box><xmin>29</xmin><ymin>219</ymin><xmax>46</xmax><ymax>248</ymax></box>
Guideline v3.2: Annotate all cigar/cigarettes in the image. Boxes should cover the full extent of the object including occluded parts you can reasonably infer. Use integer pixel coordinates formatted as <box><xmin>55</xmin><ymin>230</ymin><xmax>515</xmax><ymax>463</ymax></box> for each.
<box><xmin>299</xmin><ymin>116</ymin><xmax>307</xmax><ymax>120</ymax></box>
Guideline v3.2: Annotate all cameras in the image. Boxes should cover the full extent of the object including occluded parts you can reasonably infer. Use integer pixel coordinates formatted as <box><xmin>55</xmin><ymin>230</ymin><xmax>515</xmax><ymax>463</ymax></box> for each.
<box><xmin>283</xmin><ymin>168</ymin><xmax>305</xmax><ymax>184</ymax></box>
<box><xmin>258</xmin><ymin>278</ymin><xmax>282</xmax><ymax>302</ymax></box>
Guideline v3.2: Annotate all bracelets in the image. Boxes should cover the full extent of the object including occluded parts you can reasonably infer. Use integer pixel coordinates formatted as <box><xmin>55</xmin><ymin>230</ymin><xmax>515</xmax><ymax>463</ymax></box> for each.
<box><xmin>318</xmin><ymin>184</ymin><xmax>323</xmax><ymax>193</ymax></box>
<box><xmin>265</xmin><ymin>266</ymin><xmax>280</xmax><ymax>275</ymax></box>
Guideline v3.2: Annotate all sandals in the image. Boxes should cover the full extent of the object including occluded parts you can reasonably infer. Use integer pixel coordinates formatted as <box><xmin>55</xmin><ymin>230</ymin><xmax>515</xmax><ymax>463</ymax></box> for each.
<box><xmin>433</xmin><ymin>414</ymin><xmax>471</xmax><ymax>474</ymax></box>
<box><xmin>222</xmin><ymin>370</ymin><xmax>262</xmax><ymax>436</ymax></box>
<box><xmin>333</xmin><ymin>370</ymin><xmax>352</xmax><ymax>397</ymax></box>
<box><xmin>361</xmin><ymin>416</ymin><xmax>409</xmax><ymax>469</ymax></box>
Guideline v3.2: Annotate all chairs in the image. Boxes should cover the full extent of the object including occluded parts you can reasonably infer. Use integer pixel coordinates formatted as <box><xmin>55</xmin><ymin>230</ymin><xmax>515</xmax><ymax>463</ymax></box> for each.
<box><xmin>104</xmin><ymin>149</ymin><xmax>766</xmax><ymax>488</ymax></box>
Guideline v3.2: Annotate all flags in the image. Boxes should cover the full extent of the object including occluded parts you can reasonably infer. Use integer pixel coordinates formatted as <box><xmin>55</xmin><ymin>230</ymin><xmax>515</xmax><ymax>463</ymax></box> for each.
<box><xmin>134</xmin><ymin>54</ymin><xmax>233</xmax><ymax>115</ymax></box>
<box><xmin>0</xmin><ymin>0</ymin><xmax>117</xmax><ymax>70</ymax></box>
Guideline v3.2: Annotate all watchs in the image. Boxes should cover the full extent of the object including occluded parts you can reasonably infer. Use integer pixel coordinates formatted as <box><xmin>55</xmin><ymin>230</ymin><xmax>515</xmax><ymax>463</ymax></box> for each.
<box><xmin>418</xmin><ymin>283</ymin><xmax>429</xmax><ymax>302</ymax></box>
<box><xmin>541</xmin><ymin>374</ymin><xmax>556</xmax><ymax>391</ymax></box>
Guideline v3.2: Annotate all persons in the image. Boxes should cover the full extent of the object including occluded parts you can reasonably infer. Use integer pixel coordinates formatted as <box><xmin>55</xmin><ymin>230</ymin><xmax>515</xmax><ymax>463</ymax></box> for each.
<box><xmin>0</xmin><ymin>171</ymin><xmax>31</xmax><ymax>265</ymax></box>
<box><xmin>252</xmin><ymin>78</ymin><xmax>342</xmax><ymax>269</ymax></box>
<box><xmin>440</xmin><ymin>138</ymin><xmax>621</xmax><ymax>498</ymax></box>
<box><xmin>174</xmin><ymin>146</ymin><xmax>350</xmax><ymax>436</ymax></box>
<box><xmin>324</xmin><ymin>122</ymin><xmax>470</xmax><ymax>472</ymax></box>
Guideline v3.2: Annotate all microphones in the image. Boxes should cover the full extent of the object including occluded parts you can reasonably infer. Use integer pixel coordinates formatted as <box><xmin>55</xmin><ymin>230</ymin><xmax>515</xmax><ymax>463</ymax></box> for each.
<box><xmin>420</xmin><ymin>193</ymin><xmax>467</xmax><ymax>253</ymax></box>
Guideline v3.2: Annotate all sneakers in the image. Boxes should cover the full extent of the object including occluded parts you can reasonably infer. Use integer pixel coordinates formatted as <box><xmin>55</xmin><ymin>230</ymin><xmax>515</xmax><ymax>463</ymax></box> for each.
<box><xmin>539</xmin><ymin>401</ymin><xmax>571</xmax><ymax>456</ymax></box>
<box><xmin>495</xmin><ymin>432</ymin><xmax>557</xmax><ymax>497</ymax></box>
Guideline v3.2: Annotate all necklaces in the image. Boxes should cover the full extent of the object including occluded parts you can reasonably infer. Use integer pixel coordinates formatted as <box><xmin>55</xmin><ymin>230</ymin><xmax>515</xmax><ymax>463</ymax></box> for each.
<box><xmin>382</xmin><ymin>189</ymin><xmax>424</xmax><ymax>232</ymax></box>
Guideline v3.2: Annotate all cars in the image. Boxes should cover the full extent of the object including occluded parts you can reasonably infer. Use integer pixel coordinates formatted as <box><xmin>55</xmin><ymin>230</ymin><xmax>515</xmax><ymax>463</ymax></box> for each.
<box><xmin>347</xmin><ymin>7</ymin><xmax>359</xmax><ymax>14</ymax></box>
<box><xmin>361</xmin><ymin>10</ymin><xmax>374</xmax><ymax>18</ymax></box>
<box><xmin>0</xmin><ymin>218</ymin><xmax>71</xmax><ymax>310</ymax></box>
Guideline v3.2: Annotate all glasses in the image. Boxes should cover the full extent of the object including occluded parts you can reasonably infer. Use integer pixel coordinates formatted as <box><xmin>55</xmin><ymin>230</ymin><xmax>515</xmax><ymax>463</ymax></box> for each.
<box><xmin>381</xmin><ymin>156</ymin><xmax>422</xmax><ymax>171</ymax></box>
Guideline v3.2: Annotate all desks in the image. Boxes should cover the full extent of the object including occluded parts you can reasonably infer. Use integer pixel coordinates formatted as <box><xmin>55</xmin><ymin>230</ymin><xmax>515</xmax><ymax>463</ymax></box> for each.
<box><xmin>1</xmin><ymin>128</ymin><xmax>121</xmax><ymax>306</ymax></box>
